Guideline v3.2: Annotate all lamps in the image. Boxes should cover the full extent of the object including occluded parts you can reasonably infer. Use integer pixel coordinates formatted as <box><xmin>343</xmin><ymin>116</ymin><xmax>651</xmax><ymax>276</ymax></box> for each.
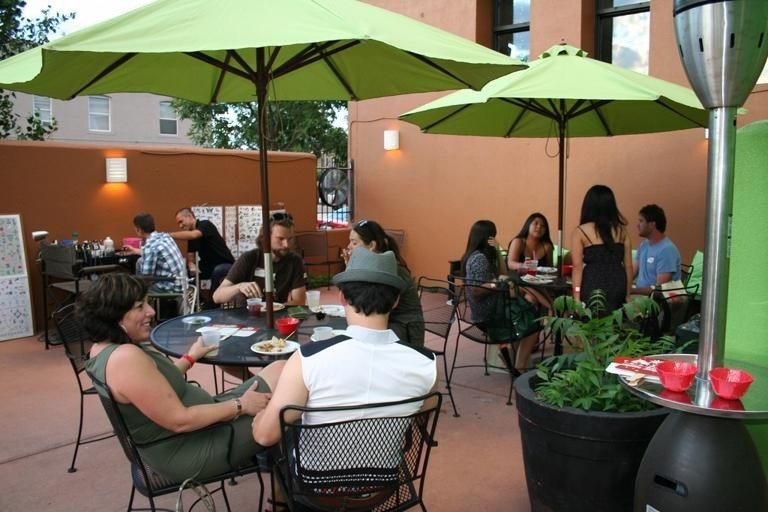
<box><xmin>383</xmin><ymin>130</ymin><xmax>399</xmax><ymax>150</ymax></box>
<box><xmin>105</xmin><ymin>157</ymin><xmax>128</xmax><ymax>183</ymax></box>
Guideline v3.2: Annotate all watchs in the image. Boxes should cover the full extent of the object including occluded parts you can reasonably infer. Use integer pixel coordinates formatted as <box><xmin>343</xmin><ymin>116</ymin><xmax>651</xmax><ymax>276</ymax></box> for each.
<box><xmin>572</xmin><ymin>286</ymin><xmax>581</xmax><ymax>293</ymax></box>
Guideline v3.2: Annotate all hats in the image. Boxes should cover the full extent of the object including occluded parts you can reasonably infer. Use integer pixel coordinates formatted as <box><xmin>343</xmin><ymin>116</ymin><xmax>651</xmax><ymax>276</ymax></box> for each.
<box><xmin>332</xmin><ymin>247</ymin><xmax>406</xmax><ymax>292</ymax></box>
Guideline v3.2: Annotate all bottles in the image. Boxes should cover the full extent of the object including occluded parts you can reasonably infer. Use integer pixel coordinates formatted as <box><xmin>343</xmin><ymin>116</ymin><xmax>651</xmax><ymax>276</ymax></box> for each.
<box><xmin>70</xmin><ymin>232</ymin><xmax>116</xmax><ymax>277</ymax></box>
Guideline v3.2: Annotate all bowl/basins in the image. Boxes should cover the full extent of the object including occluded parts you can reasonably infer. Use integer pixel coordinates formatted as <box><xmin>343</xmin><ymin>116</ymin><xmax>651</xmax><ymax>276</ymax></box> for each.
<box><xmin>708</xmin><ymin>367</ymin><xmax>754</xmax><ymax>401</ymax></box>
<box><xmin>562</xmin><ymin>265</ymin><xmax>572</xmax><ymax>273</ymax></box>
<box><xmin>709</xmin><ymin>395</ymin><xmax>744</xmax><ymax>411</ymax></box>
<box><xmin>657</xmin><ymin>387</ymin><xmax>691</xmax><ymax>406</ymax></box>
<box><xmin>274</xmin><ymin>318</ymin><xmax>300</xmax><ymax>335</ymax></box>
<box><xmin>655</xmin><ymin>361</ymin><xmax>696</xmax><ymax>392</ymax></box>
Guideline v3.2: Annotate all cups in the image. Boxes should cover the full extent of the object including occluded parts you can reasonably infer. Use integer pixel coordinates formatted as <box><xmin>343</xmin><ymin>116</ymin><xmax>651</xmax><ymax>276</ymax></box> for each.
<box><xmin>527</xmin><ymin>259</ymin><xmax>538</xmax><ymax>270</ymax></box>
<box><xmin>312</xmin><ymin>326</ymin><xmax>332</xmax><ymax>341</ymax></box>
<box><xmin>305</xmin><ymin>291</ymin><xmax>320</xmax><ymax>309</ymax></box>
<box><xmin>247</xmin><ymin>298</ymin><xmax>262</xmax><ymax>316</ymax></box>
<box><xmin>201</xmin><ymin>330</ymin><xmax>220</xmax><ymax>357</ymax></box>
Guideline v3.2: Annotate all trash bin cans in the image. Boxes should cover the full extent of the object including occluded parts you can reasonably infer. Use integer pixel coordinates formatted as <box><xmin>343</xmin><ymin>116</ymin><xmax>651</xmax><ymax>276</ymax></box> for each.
<box><xmin>447</xmin><ymin>260</ymin><xmax>463</xmax><ymax>299</ymax></box>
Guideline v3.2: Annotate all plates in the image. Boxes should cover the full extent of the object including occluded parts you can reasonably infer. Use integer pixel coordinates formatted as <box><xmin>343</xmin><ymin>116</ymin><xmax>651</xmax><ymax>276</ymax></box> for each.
<box><xmin>520</xmin><ymin>266</ymin><xmax>559</xmax><ymax>285</ymax></box>
<box><xmin>309</xmin><ymin>330</ymin><xmax>346</xmax><ymax>342</ymax></box>
<box><xmin>251</xmin><ymin>340</ymin><xmax>300</xmax><ymax>355</ymax></box>
<box><xmin>311</xmin><ymin>304</ymin><xmax>345</xmax><ymax>316</ymax></box>
<box><xmin>181</xmin><ymin>315</ymin><xmax>211</xmax><ymax>325</ymax></box>
<box><xmin>245</xmin><ymin>301</ymin><xmax>284</xmax><ymax>312</ymax></box>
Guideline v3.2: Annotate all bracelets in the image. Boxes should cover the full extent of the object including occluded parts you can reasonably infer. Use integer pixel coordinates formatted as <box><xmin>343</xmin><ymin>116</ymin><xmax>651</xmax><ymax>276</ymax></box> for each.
<box><xmin>180</xmin><ymin>354</ymin><xmax>195</xmax><ymax>368</ymax></box>
<box><xmin>232</xmin><ymin>397</ymin><xmax>242</xmax><ymax>422</ymax></box>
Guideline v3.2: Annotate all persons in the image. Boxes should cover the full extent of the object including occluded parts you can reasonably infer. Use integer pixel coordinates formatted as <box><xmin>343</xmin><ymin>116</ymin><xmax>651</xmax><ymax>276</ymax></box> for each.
<box><xmin>507</xmin><ymin>211</ymin><xmax>556</xmax><ymax>355</ymax></box>
<box><xmin>212</xmin><ymin>211</ymin><xmax>309</xmax><ymax>382</ymax></box>
<box><xmin>78</xmin><ymin>271</ymin><xmax>303</xmax><ymax>484</ymax></box>
<box><xmin>630</xmin><ymin>203</ymin><xmax>681</xmax><ymax>296</ymax></box>
<box><xmin>339</xmin><ymin>219</ymin><xmax>425</xmax><ymax>452</ymax></box>
<box><xmin>249</xmin><ymin>245</ymin><xmax>438</xmax><ymax>512</ymax></box>
<box><xmin>568</xmin><ymin>183</ymin><xmax>633</xmax><ymax>331</ymax></box>
<box><xmin>169</xmin><ymin>207</ymin><xmax>236</xmax><ymax>309</ymax></box>
<box><xmin>462</xmin><ymin>219</ymin><xmax>545</xmax><ymax>380</ymax></box>
<box><xmin>122</xmin><ymin>212</ymin><xmax>188</xmax><ymax>294</ymax></box>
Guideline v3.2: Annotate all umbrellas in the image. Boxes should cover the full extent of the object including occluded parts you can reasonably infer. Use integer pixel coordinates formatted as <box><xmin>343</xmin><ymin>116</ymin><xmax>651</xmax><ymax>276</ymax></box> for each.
<box><xmin>0</xmin><ymin>0</ymin><xmax>531</xmax><ymax>329</ymax></box>
<box><xmin>399</xmin><ymin>54</ymin><xmax>751</xmax><ymax>354</ymax></box>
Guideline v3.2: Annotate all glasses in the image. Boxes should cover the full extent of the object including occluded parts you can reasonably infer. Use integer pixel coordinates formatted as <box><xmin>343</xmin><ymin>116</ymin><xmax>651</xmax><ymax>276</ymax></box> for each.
<box><xmin>358</xmin><ymin>218</ymin><xmax>367</xmax><ymax>226</ymax></box>
<box><xmin>270</xmin><ymin>212</ymin><xmax>294</xmax><ymax>221</ymax></box>
